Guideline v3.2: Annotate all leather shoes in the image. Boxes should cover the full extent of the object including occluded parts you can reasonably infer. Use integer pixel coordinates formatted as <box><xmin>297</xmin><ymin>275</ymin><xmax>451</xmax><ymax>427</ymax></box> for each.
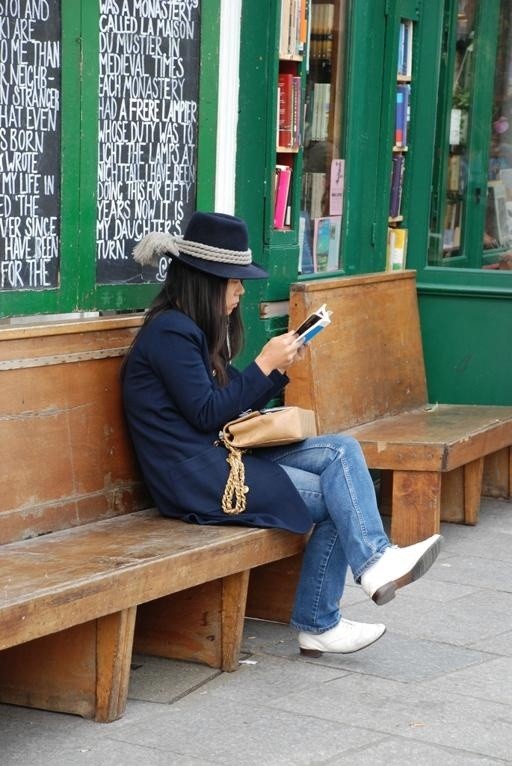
<box><xmin>297</xmin><ymin>617</ymin><xmax>388</xmax><ymax>659</ymax></box>
<box><xmin>358</xmin><ymin>532</ymin><xmax>443</xmax><ymax>606</ymax></box>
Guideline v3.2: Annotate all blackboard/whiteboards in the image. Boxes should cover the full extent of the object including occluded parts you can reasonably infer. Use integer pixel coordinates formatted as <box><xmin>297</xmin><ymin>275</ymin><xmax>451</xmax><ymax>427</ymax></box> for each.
<box><xmin>0</xmin><ymin>1</ymin><xmax>81</xmax><ymax>312</ymax></box>
<box><xmin>81</xmin><ymin>0</ymin><xmax>217</xmax><ymax>312</ymax></box>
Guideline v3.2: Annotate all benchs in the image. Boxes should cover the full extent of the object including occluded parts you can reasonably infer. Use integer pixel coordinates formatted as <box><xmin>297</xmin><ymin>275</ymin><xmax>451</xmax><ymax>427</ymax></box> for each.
<box><xmin>288</xmin><ymin>271</ymin><xmax>509</xmax><ymax>549</ymax></box>
<box><xmin>0</xmin><ymin>312</ymin><xmax>316</xmax><ymax>722</ymax></box>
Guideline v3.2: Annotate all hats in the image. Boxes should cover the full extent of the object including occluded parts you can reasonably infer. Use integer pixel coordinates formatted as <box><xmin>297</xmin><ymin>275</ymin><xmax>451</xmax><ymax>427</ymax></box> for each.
<box><xmin>131</xmin><ymin>212</ymin><xmax>270</xmax><ymax>281</ymax></box>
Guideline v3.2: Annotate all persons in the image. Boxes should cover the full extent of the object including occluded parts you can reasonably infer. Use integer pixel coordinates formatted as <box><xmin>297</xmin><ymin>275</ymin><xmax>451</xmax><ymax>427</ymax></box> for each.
<box><xmin>118</xmin><ymin>211</ymin><xmax>443</xmax><ymax>660</ymax></box>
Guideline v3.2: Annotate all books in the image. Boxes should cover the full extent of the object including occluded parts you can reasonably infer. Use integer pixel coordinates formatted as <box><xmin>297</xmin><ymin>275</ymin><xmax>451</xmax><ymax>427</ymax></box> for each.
<box><xmin>387</xmin><ymin>20</ymin><xmax>412</xmax><ymax>272</ymax></box>
<box><xmin>289</xmin><ymin>302</ymin><xmax>336</xmax><ymax>350</ymax></box>
<box><xmin>274</xmin><ymin>0</ymin><xmax>340</xmax><ymax>273</ymax></box>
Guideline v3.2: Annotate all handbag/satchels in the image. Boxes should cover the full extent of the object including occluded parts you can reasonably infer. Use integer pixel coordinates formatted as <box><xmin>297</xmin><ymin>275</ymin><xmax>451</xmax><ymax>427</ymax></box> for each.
<box><xmin>214</xmin><ymin>404</ymin><xmax>320</xmax><ymax>515</ymax></box>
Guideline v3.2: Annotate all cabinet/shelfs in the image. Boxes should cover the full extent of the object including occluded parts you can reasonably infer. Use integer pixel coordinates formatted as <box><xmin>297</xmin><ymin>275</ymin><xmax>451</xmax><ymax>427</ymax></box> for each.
<box><xmin>266</xmin><ymin>1</ymin><xmax>500</xmax><ymax>268</ymax></box>
